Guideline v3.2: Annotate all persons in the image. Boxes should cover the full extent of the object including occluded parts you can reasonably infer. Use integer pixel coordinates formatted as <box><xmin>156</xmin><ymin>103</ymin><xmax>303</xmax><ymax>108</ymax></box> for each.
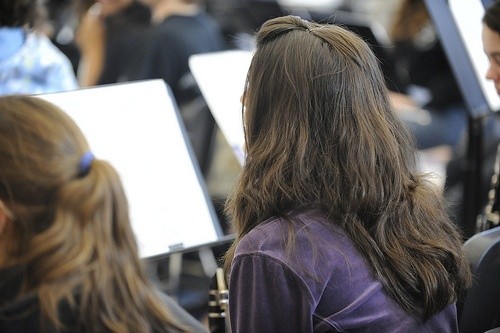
<box><xmin>1</xmin><ymin>94</ymin><xmax>212</xmax><ymax>333</ymax></box>
<box><xmin>228</xmin><ymin>16</ymin><xmax>474</xmax><ymax>333</ymax></box>
<box><xmin>480</xmin><ymin>1</ymin><xmax>500</xmax><ymax>97</ymax></box>
<box><xmin>0</xmin><ymin>0</ymin><xmax>315</xmax><ymax>105</ymax></box>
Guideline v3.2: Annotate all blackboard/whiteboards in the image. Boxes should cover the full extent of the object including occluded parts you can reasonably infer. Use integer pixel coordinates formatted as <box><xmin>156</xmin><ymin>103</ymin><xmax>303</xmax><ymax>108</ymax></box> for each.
<box><xmin>28</xmin><ymin>78</ymin><xmax>225</xmax><ymax>264</ymax></box>
<box><xmin>426</xmin><ymin>0</ymin><xmax>500</xmax><ymax>120</ymax></box>
<box><xmin>189</xmin><ymin>48</ymin><xmax>259</xmax><ymax>169</ymax></box>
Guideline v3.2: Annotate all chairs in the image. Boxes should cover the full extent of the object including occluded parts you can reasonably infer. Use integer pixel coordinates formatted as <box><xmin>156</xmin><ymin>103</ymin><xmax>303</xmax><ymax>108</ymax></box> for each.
<box><xmin>175</xmin><ymin>75</ymin><xmax>243</xmax><ymax>183</ymax></box>
